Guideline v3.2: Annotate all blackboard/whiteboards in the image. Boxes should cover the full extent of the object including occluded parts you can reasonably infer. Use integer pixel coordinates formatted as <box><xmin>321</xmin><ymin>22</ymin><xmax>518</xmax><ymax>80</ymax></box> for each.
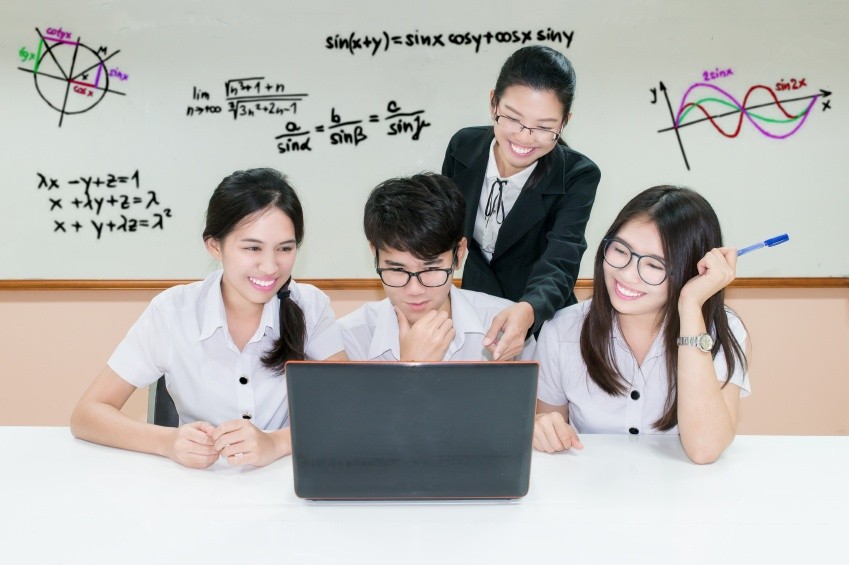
<box><xmin>0</xmin><ymin>1</ymin><xmax>849</xmax><ymax>290</ymax></box>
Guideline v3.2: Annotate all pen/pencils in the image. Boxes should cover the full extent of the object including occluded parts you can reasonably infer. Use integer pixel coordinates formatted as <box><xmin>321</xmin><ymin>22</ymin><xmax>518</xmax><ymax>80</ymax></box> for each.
<box><xmin>737</xmin><ymin>233</ymin><xmax>790</xmax><ymax>256</ymax></box>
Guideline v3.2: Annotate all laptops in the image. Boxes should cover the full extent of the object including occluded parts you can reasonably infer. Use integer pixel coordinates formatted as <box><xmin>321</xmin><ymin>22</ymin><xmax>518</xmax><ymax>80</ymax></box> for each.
<box><xmin>284</xmin><ymin>360</ymin><xmax>539</xmax><ymax>501</ymax></box>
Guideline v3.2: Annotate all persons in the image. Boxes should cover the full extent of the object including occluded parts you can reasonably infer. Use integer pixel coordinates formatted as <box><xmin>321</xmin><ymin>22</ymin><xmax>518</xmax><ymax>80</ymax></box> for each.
<box><xmin>441</xmin><ymin>46</ymin><xmax>602</xmax><ymax>362</ymax></box>
<box><xmin>333</xmin><ymin>174</ymin><xmax>537</xmax><ymax>362</ymax></box>
<box><xmin>532</xmin><ymin>184</ymin><xmax>752</xmax><ymax>466</ymax></box>
<box><xmin>69</xmin><ymin>168</ymin><xmax>350</xmax><ymax>470</ymax></box>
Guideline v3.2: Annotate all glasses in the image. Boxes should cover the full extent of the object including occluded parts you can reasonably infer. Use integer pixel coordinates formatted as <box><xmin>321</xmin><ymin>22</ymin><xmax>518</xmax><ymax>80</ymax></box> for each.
<box><xmin>600</xmin><ymin>233</ymin><xmax>673</xmax><ymax>286</ymax></box>
<box><xmin>371</xmin><ymin>248</ymin><xmax>464</xmax><ymax>288</ymax></box>
<box><xmin>493</xmin><ymin>100</ymin><xmax>567</xmax><ymax>144</ymax></box>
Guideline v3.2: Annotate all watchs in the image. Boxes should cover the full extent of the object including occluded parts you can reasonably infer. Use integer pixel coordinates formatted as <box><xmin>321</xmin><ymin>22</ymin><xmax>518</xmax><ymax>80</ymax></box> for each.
<box><xmin>676</xmin><ymin>334</ymin><xmax>714</xmax><ymax>353</ymax></box>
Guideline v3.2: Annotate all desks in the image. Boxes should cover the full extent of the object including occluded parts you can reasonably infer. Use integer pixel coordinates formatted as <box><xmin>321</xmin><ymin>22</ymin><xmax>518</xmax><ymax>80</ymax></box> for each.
<box><xmin>0</xmin><ymin>427</ymin><xmax>849</xmax><ymax>565</ymax></box>
